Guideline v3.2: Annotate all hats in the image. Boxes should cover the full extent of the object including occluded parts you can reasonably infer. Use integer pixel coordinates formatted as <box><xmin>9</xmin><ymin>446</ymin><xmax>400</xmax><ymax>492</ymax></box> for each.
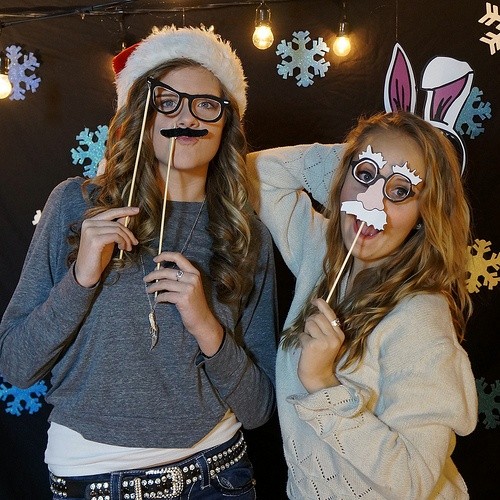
<box><xmin>111</xmin><ymin>22</ymin><xmax>248</xmax><ymax>122</ymax></box>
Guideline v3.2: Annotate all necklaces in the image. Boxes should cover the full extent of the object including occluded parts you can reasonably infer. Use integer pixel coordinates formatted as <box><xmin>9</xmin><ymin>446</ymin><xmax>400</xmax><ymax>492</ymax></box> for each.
<box><xmin>133</xmin><ymin>194</ymin><xmax>209</xmax><ymax>351</ymax></box>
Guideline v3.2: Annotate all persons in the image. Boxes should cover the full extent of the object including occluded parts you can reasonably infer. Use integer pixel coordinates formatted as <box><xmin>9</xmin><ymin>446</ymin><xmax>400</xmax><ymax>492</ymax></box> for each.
<box><xmin>1</xmin><ymin>24</ymin><xmax>280</xmax><ymax>499</ymax></box>
<box><xmin>245</xmin><ymin>108</ymin><xmax>478</xmax><ymax>500</ymax></box>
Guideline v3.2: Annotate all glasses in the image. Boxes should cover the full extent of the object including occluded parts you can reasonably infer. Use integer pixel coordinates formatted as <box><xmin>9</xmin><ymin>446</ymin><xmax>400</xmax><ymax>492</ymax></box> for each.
<box><xmin>348</xmin><ymin>157</ymin><xmax>424</xmax><ymax>203</ymax></box>
<box><xmin>143</xmin><ymin>74</ymin><xmax>232</xmax><ymax>123</ymax></box>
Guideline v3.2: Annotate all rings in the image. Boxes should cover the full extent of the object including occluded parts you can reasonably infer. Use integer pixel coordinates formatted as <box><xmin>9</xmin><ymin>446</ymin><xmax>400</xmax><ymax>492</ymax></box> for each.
<box><xmin>330</xmin><ymin>317</ymin><xmax>341</xmax><ymax>328</ymax></box>
<box><xmin>176</xmin><ymin>269</ymin><xmax>184</xmax><ymax>281</ymax></box>
